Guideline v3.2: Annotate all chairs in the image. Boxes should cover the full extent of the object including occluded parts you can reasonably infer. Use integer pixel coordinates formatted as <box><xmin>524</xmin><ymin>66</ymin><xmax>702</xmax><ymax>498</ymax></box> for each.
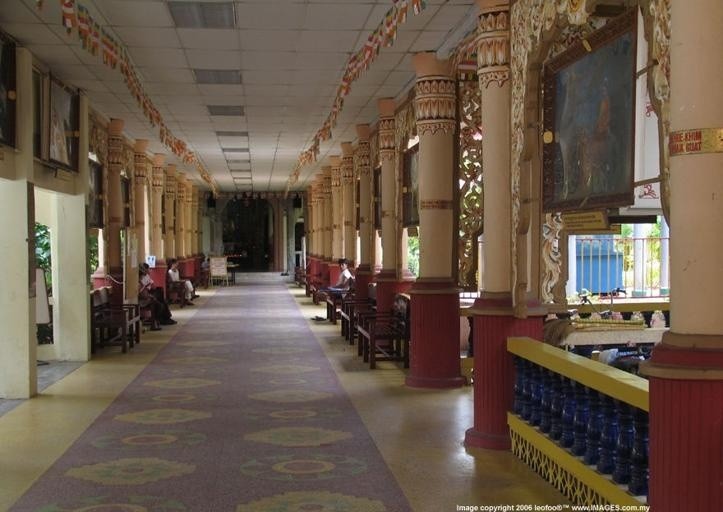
<box><xmin>89</xmin><ymin>277</ymin><xmax>198</xmax><ymax>353</ymax></box>
<box><xmin>304</xmin><ymin>273</ymin><xmax>411</xmax><ymax>371</ymax></box>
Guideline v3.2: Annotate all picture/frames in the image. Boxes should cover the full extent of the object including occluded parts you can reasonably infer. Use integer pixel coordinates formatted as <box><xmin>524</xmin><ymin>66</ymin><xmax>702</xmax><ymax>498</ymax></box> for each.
<box><xmin>541</xmin><ymin>5</ymin><xmax>636</xmax><ymax>213</ymax></box>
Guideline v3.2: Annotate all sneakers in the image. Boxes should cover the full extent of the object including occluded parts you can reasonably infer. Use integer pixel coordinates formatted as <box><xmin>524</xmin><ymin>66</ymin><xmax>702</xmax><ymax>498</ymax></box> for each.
<box><xmin>161</xmin><ymin>319</ymin><xmax>177</xmax><ymax>324</ymax></box>
<box><xmin>186</xmin><ymin>300</ymin><xmax>195</xmax><ymax>305</ymax></box>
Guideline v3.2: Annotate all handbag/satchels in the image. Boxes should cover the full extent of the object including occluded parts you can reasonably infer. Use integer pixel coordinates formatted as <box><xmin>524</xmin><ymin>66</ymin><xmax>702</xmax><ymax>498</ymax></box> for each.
<box><xmin>141</xmin><ymin>288</ymin><xmax>150</xmax><ymax>298</ymax></box>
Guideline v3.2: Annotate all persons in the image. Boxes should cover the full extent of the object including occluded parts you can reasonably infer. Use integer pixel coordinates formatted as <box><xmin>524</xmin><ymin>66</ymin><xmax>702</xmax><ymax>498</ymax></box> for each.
<box><xmin>169</xmin><ymin>259</ymin><xmax>199</xmax><ymax>305</ymax></box>
<box><xmin>327</xmin><ymin>259</ymin><xmax>353</xmax><ymax>300</ymax></box>
<box><xmin>139</xmin><ymin>263</ymin><xmax>177</xmax><ymax>325</ymax></box>
